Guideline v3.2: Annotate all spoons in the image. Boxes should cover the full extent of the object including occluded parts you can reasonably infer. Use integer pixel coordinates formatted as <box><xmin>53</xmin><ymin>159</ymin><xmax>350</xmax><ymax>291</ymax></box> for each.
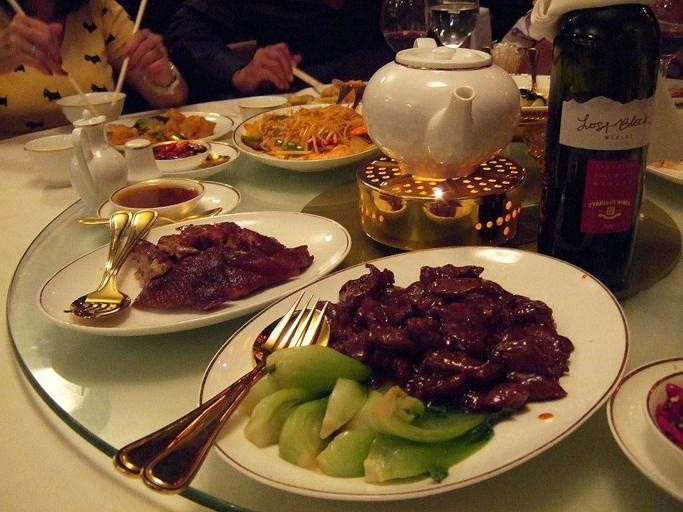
<box><xmin>113</xmin><ymin>310</ymin><xmax>329</xmax><ymax>479</ymax></box>
<box><xmin>65</xmin><ymin>210</ymin><xmax>132</xmax><ymax>324</ymax></box>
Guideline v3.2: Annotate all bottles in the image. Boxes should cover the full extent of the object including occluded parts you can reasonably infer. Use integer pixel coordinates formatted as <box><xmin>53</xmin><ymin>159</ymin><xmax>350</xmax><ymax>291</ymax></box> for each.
<box><xmin>536</xmin><ymin>4</ymin><xmax>662</xmax><ymax>290</ymax></box>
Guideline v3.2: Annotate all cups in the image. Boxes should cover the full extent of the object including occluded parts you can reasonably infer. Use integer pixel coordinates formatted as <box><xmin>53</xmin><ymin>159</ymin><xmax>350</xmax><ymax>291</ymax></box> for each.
<box><xmin>238</xmin><ymin>95</ymin><xmax>288</xmax><ymax>119</ymax></box>
<box><xmin>123</xmin><ymin>133</ymin><xmax>158</xmax><ymax>178</ymax></box>
<box><xmin>380</xmin><ymin>0</ymin><xmax>479</xmax><ymax>54</ymax></box>
<box><xmin>26</xmin><ymin>135</ymin><xmax>76</xmax><ymax>188</ymax></box>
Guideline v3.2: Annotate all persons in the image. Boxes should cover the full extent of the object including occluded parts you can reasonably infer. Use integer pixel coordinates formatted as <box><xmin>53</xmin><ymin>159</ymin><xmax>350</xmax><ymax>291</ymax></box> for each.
<box><xmin>159</xmin><ymin>0</ymin><xmax>395</xmax><ymax>100</ymax></box>
<box><xmin>0</xmin><ymin>0</ymin><xmax>190</xmax><ymax>143</ymax></box>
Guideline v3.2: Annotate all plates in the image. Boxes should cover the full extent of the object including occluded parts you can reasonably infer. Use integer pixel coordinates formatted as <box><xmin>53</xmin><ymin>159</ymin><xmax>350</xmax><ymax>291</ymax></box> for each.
<box><xmin>233</xmin><ymin>101</ymin><xmax>380</xmax><ymax>170</ymax></box>
<box><xmin>105</xmin><ymin>113</ymin><xmax>234</xmax><ymax>141</ymax></box>
<box><xmin>108</xmin><ymin>178</ymin><xmax>206</xmax><ymax>218</ymax></box>
<box><xmin>196</xmin><ymin>246</ymin><xmax>631</xmax><ymax>503</ymax></box>
<box><xmin>158</xmin><ymin>140</ymin><xmax>239</xmax><ymax>182</ymax></box>
<box><xmin>608</xmin><ymin>357</ymin><xmax>683</xmax><ymax>506</ymax></box>
<box><xmin>146</xmin><ymin>139</ymin><xmax>212</xmax><ymax>171</ymax></box>
<box><xmin>40</xmin><ymin>209</ymin><xmax>353</xmax><ymax>340</ymax></box>
<box><xmin>94</xmin><ymin>181</ymin><xmax>244</xmax><ymax>231</ymax></box>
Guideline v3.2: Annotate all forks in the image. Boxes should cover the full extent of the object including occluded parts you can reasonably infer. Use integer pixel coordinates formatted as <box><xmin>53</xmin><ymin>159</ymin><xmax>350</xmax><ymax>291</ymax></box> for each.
<box><xmin>62</xmin><ymin>209</ymin><xmax>161</xmax><ymax>318</ymax></box>
<box><xmin>143</xmin><ymin>291</ymin><xmax>330</xmax><ymax>493</ymax></box>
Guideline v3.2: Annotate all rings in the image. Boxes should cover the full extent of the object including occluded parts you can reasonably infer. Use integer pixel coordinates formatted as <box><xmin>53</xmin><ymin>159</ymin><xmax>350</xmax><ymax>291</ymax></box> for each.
<box><xmin>31</xmin><ymin>43</ymin><xmax>36</xmax><ymax>56</ymax></box>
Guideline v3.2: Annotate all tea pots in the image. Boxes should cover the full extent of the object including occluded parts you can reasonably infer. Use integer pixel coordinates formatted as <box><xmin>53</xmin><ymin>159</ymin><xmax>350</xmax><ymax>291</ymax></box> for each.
<box><xmin>362</xmin><ymin>39</ymin><xmax>521</xmax><ymax>180</ymax></box>
<box><xmin>69</xmin><ymin>106</ymin><xmax>127</xmax><ymax>213</ymax></box>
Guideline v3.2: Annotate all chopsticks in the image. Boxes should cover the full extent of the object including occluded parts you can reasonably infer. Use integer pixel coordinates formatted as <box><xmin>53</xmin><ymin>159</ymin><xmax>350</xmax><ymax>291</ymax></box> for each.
<box><xmin>292</xmin><ymin>65</ymin><xmax>325</xmax><ymax>93</ymax></box>
<box><xmin>8</xmin><ymin>0</ymin><xmax>147</xmax><ymax>121</ymax></box>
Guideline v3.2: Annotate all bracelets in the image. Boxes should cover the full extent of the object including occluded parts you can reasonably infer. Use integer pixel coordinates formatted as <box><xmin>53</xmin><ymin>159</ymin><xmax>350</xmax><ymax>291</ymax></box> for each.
<box><xmin>144</xmin><ymin>62</ymin><xmax>182</xmax><ymax>97</ymax></box>
